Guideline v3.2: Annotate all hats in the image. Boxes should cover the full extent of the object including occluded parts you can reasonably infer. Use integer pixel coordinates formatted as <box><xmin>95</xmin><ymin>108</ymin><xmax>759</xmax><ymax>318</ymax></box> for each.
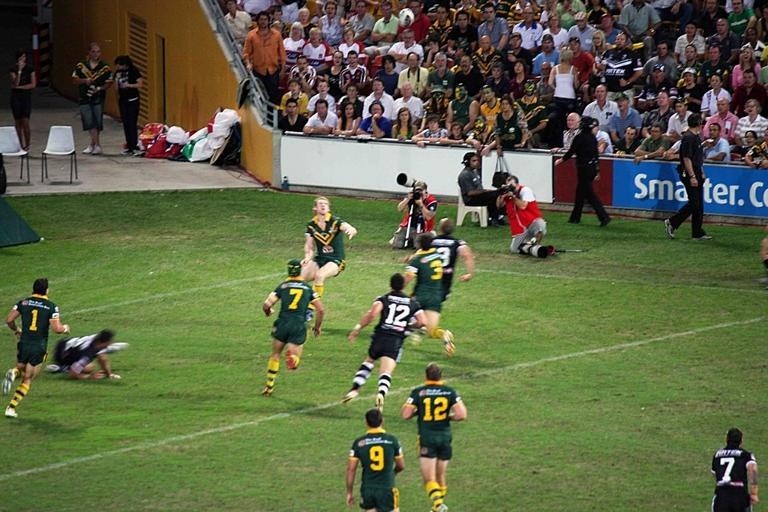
<box><xmin>574</xmin><ymin>11</ymin><xmax>586</xmax><ymax>20</ymax></box>
<box><xmin>413</xmin><ymin>180</ymin><xmax>427</xmax><ymax>191</ymax></box>
<box><xmin>682</xmin><ymin>66</ymin><xmax>696</xmax><ymax>76</ymax></box>
<box><xmin>652</xmin><ymin>63</ymin><xmax>664</xmax><ymax>72</ymax></box>
<box><xmin>614</xmin><ymin>94</ymin><xmax>628</xmax><ymax>100</ymax></box>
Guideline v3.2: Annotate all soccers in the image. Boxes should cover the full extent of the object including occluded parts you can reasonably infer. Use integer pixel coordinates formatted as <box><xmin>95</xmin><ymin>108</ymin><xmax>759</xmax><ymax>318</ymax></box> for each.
<box><xmin>399</xmin><ymin>9</ymin><xmax>416</xmax><ymax>27</ymax></box>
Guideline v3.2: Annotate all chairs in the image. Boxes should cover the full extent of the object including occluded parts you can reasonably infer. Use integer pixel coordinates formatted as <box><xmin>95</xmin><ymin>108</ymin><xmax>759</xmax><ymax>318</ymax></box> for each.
<box><xmin>0</xmin><ymin>126</ymin><xmax>31</xmax><ymax>184</ymax></box>
<box><xmin>41</xmin><ymin>125</ymin><xmax>78</xmax><ymax>185</ymax></box>
<box><xmin>455</xmin><ymin>183</ymin><xmax>488</xmax><ymax>229</ymax></box>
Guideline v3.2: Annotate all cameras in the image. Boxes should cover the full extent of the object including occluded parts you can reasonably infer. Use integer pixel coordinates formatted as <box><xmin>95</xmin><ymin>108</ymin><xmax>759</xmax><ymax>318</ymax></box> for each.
<box><xmin>497</xmin><ymin>183</ymin><xmax>516</xmax><ymax>195</ymax></box>
<box><xmin>413</xmin><ymin>190</ymin><xmax>422</xmax><ymax>200</ymax></box>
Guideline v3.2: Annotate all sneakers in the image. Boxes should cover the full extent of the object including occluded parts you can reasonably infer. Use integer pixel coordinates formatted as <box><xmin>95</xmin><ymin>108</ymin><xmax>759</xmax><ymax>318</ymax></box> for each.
<box><xmin>693</xmin><ymin>235</ymin><xmax>712</xmax><ymax>241</ymax></box>
<box><xmin>443</xmin><ymin>330</ymin><xmax>455</xmax><ymax>358</ymax></box>
<box><xmin>285</xmin><ymin>351</ymin><xmax>293</xmax><ymax>369</ymax></box>
<box><xmin>341</xmin><ymin>390</ymin><xmax>358</xmax><ymax>405</ymax></box>
<box><xmin>2</xmin><ymin>370</ymin><xmax>15</xmax><ymax>395</ymax></box>
<box><xmin>92</xmin><ymin>147</ymin><xmax>102</xmax><ymax>154</ymax></box>
<box><xmin>82</xmin><ymin>146</ymin><xmax>92</xmax><ymax>154</ymax></box>
<box><xmin>664</xmin><ymin>219</ymin><xmax>675</xmax><ymax>239</ymax></box>
<box><xmin>375</xmin><ymin>392</ymin><xmax>384</xmax><ymax>413</ymax></box>
<box><xmin>5</xmin><ymin>406</ymin><xmax>18</xmax><ymax>418</ymax></box>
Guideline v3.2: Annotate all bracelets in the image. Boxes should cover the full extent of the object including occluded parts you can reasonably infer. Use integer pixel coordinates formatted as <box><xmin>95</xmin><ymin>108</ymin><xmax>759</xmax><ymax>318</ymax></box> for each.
<box><xmin>354</xmin><ymin>324</ymin><xmax>362</xmax><ymax>331</ymax></box>
<box><xmin>597</xmin><ymin>169</ymin><xmax>600</xmax><ymax>172</ymax></box>
<box><xmin>690</xmin><ymin>175</ymin><xmax>695</xmax><ymax>178</ymax></box>
<box><xmin>420</xmin><ymin>204</ymin><xmax>424</xmax><ymax>210</ymax></box>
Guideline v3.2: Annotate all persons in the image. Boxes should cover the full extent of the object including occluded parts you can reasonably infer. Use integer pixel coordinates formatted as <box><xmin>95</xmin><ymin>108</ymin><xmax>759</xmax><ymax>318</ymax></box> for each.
<box><xmin>555</xmin><ymin>117</ymin><xmax>611</xmax><ymax>227</ymax></box>
<box><xmin>401</xmin><ymin>362</ymin><xmax>468</xmax><ymax>512</ymax></box>
<box><xmin>297</xmin><ymin>196</ymin><xmax>358</xmax><ymax>298</ymax></box>
<box><xmin>1</xmin><ymin>278</ymin><xmax>71</xmax><ymax>418</ymax></box>
<box><xmin>262</xmin><ymin>259</ymin><xmax>324</xmax><ymax>398</ymax></box>
<box><xmin>343</xmin><ymin>218</ymin><xmax>475</xmax><ymax>414</ymax></box>
<box><xmin>664</xmin><ymin>114</ymin><xmax>712</xmax><ymax>241</ymax></box>
<box><xmin>391</xmin><ymin>182</ymin><xmax>439</xmax><ymax>249</ymax></box>
<box><xmin>346</xmin><ymin>409</ymin><xmax>405</xmax><ymax>512</ymax></box>
<box><xmin>711</xmin><ymin>427</ymin><xmax>759</xmax><ymax>512</ymax></box>
<box><xmin>496</xmin><ymin>176</ymin><xmax>554</xmax><ymax>259</ymax></box>
<box><xmin>759</xmin><ymin>225</ymin><xmax>768</xmax><ymax>291</ymax></box>
<box><xmin>46</xmin><ymin>329</ymin><xmax>130</xmax><ymax>380</ymax></box>
<box><xmin>458</xmin><ymin>153</ymin><xmax>507</xmax><ymax>226</ymax></box>
<box><xmin>72</xmin><ymin>43</ymin><xmax>113</xmax><ymax>155</ymax></box>
<box><xmin>9</xmin><ymin>48</ymin><xmax>37</xmax><ymax>158</ymax></box>
<box><xmin>113</xmin><ymin>55</ymin><xmax>145</xmax><ymax>157</ymax></box>
<box><xmin>215</xmin><ymin>2</ymin><xmax>767</xmax><ymax>170</ymax></box>
